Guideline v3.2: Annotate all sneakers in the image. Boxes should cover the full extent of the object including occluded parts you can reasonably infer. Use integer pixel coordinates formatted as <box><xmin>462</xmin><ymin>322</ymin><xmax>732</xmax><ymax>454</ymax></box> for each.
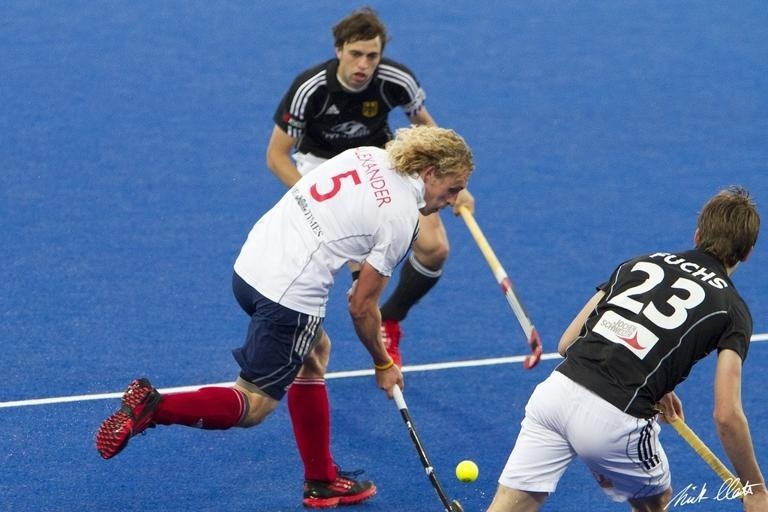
<box><xmin>378</xmin><ymin>316</ymin><xmax>404</xmax><ymax>372</ymax></box>
<box><xmin>94</xmin><ymin>377</ymin><xmax>164</xmax><ymax>462</ymax></box>
<box><xmin>301</xmin><ymin>471</ymin><xmax>382</xmax><ymax>509</ymax></box>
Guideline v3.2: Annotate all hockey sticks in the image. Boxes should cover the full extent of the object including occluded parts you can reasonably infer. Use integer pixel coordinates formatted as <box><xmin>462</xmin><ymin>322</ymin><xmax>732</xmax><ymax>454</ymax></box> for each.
<box><xmin>457</xmin><ymin>202</ymin><xmax>544</xmax><ymax>368</ymax></box>
<box><xmin>392</xmin><ymin>384</ymin><xmax>463</xmax><ymax>511</ymax></box>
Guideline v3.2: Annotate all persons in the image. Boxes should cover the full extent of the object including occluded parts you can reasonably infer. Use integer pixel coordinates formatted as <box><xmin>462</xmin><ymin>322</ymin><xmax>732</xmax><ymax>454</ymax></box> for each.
<box><xmin>485</xmin><ymin>184</ymin><xmax>768</xmax><ymax>512</ymax></box>
<box><xmin>95</xmin><ymin>125</ymin><xmax>475</xmax><ymax>508</ymax></box>
<box><xmin>265</xmin><ymin>6</ymin><xmax>474</xmax><ymax>370</ymax></box>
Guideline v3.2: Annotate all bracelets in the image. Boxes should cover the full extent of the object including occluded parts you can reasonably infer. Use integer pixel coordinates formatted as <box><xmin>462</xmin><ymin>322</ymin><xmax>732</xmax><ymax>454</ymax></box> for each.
<box><xmin>375</xmin><ymin>357</ymin><xmax>394</xmax><ymax>370</ymax></box>
